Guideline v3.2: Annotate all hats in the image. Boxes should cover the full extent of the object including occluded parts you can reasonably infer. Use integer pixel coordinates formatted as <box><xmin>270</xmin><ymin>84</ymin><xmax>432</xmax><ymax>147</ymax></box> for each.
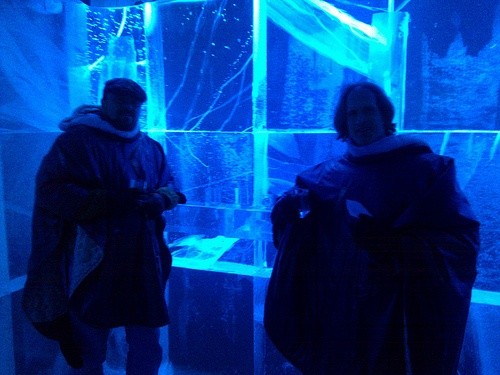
<box><xmin>104</xmin><ymin>78</ymin><xmax>146</xmax><ymax>103</ymax></box>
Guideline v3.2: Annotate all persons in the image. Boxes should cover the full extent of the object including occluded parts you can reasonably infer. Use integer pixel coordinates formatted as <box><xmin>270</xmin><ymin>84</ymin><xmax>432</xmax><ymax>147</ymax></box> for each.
<box><xmin>270</xmin><ymin>82</ymin><xmax>480</xmax><ymax>375</ymax></box>
<box><xmin>59</xmin><ymin>79</ymin><xmax>184</xmax><ymax>375</ymax></box>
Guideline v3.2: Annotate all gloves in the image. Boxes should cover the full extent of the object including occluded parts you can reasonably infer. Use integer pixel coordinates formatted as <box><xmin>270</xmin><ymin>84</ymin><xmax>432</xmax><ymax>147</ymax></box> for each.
<box><xmin>349</xmin><ymin>211</ymin><xmax>400</xmax><ymax>256</ymax></box>
<box><xmin>135</xmin><ymin>187</ymin><xmax>179</xmax><ymax>215</ymax></box>
